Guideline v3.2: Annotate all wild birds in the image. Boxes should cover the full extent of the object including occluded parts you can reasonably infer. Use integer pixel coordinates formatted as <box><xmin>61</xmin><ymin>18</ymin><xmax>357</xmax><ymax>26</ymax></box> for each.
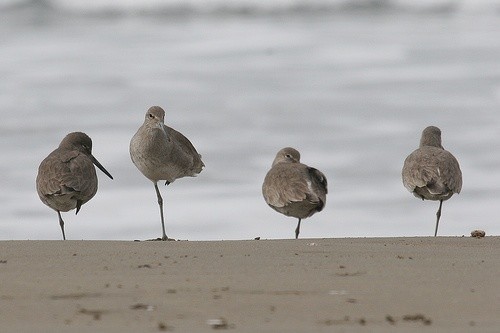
<box><xmin>35</xmin><ymin>132</ymin><xmax>113</xmax><ymax>241</ymax></box>
<box><xmin>402</xmin><ymin>126</ymin><xmax>462</xmax><ymax>237</ymax></box>
<box><xmin>261</xmin><ymin>147</ymin><xmax>327</xmax><ymax>239</ymax></box>
<box><xmin>129</xmin><ymin>106</ymin><xmax>205</xmax><ymax>238</ymax></box>
